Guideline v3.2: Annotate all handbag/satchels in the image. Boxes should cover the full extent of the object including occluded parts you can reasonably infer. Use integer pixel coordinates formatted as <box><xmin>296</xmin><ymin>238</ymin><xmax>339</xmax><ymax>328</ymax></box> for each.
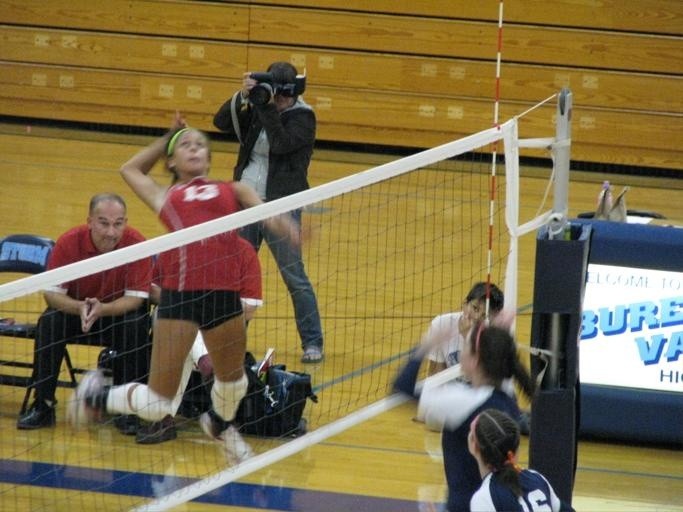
<box><xmin>177</xmin><ymin>351</ymin><xmax>317</xmax><ymax>438</ymax></box>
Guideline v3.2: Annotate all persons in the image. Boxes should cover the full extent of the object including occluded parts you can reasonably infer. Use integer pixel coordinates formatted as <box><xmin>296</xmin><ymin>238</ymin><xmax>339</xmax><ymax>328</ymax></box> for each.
<box><xmin>392</xmin><ymin>327</ymin><xmax>533</xmax><ymax>511</ymax></box>
<box><xmin>16</xmin><ymin>191</ymin><xmax>158</xmax><ymax>436</ymax></box>
<box><xmin>146</xmin><ymin>233</ymin><xmax>264</xmax><ymax>378</ymax></box>
<box><xmin>212</xmin><ymin>63</ymin><xmax>324</xmax><ymax>365</ymax></box>
<box><xmin>66</xmin><ymin>109</ymin><xmax>300</xmax><ymax>464</ymax></box>
<box><xmin>407</xmin><ymin>280</ymin><xmax>507</xmax><ymax>403</ymax></box>
<box><xmin>469</xmin><ymin>409</ymin><xmax>578</xmax><ymax>511</ymax></box>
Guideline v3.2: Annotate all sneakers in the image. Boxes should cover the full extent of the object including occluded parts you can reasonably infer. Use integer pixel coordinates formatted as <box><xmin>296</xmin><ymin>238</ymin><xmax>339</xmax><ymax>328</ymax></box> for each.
<box><xmin>69</xmin><ymin>370</ymin><xmax>104</xmax><ymax>429</ymax></box>
<box><xmin>199</xmin><ymin>410</ymin><xmax>252</xmax><ymax>465</ymax></box>
<box><xmin>17</xmin><ymin>400</ymin><xmax>56</xmax><ymax>429</ymax></box>
<box><xmin>113</xmin><ymin>415</ymin><xmax>139</xmax><ymax>435</ymax></box>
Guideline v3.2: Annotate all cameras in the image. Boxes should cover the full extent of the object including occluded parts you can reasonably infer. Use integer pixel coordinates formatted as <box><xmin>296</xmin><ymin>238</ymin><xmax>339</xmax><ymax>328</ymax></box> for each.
<box><xmin>249</xmin><ymin>72</ymin><xmax>305</xmax><ymax>106</ymax></box>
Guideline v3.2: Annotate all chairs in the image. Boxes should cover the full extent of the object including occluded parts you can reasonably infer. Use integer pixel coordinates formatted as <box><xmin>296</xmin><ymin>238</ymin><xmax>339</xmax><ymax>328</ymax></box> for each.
<box><xmin>0</xmin><ymin>235</ymin><xmax>55</xmax><ymax>415</ymax></box>
<box><xmin>55</xmin><ymin>327</ymin><xmax>114</xmax><ymax>388</ymax></box>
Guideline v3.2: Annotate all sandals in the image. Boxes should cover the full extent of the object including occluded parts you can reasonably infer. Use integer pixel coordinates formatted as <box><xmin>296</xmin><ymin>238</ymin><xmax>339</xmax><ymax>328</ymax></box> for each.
<box><xmin>301</xmin><ymin>347</ymin><xmax>322</xmax><ymax>363</ymax></box>
